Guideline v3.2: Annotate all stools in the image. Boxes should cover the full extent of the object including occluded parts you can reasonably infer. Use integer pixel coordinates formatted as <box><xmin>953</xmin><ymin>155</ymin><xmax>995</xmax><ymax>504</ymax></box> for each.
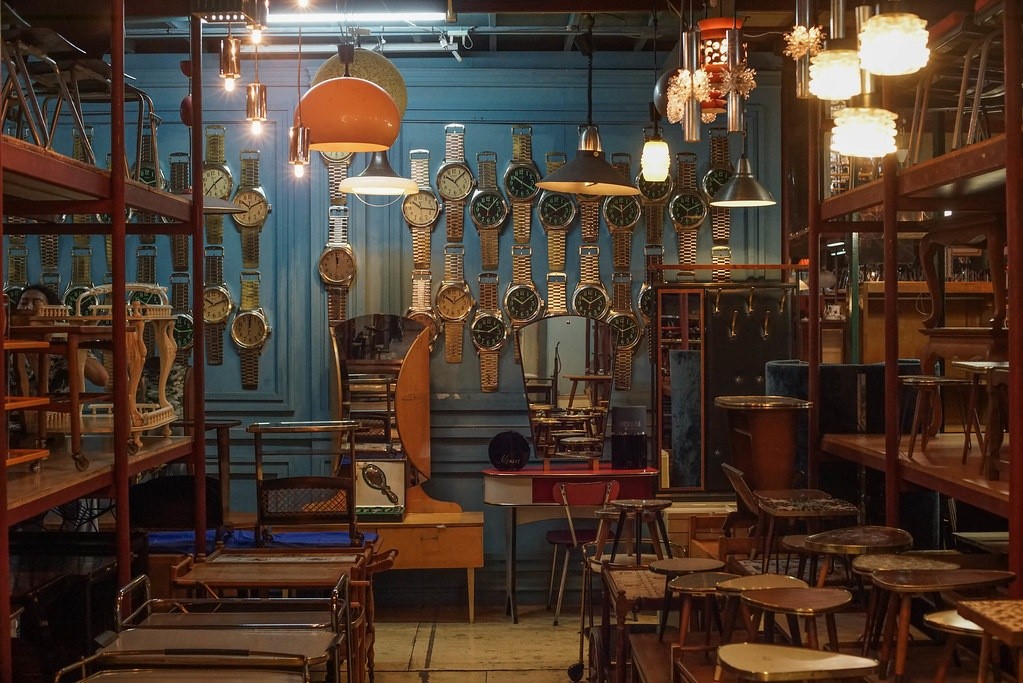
<box><xmin>567</xmin><ymin>375</ymin><xmax>613</xmax><ymax>415</ymax></box>
<box><xmin>906</xmin><ymin>8</ymin><xmax>1003</xmax><ymax>169</ymax></box>
<box><xmin>530</xmin><ymin>402</ymin><xmax>607</xmax><ymax>458</ymax></box>
<box><xmin>899</xmin><ymin>375</ymin><xmax>985</xmax><ymax>459</ymax></box>
<box><xmin>595</xmin><ymin>488</ymin><xmax>1023</xmax><ymax>683</ymax></box>
<box><xmin>951</xmin><ymin>361</ymin><xmax>1010</xmax><ymax>475</ymax></box>
<box><xmin>0</xmin><ymin>1</ymin><xmax>163</xmax><ymax>191</ymax></box>
<box><xmin>965</xmin><ymin>0</ymin><xmax>1005</xmax><ymax>146</ymax></box>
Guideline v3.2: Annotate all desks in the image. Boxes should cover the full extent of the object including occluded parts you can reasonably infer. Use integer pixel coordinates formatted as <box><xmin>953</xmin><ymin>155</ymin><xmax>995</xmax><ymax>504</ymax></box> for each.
<box><xmin>348</xmin><ymin>372</ymin><xmax>396</xmax><ymax>444</ymax></box>
<box><xmin>917</xmin><ymin>324</ymin><xmax>1010</xmax><ymax>442</ymax></box>
<box><xmin>10</xmin><ymin>325</ymin><xmax>140</xmax><ymax>473</ymax></box>
<box><xmin>845</xmin><ymin>281</ymin><xmax>994</xmax><ymax>434</ymax></box>
<box><xmin>246</xmin><ymin>421</ymin><xmax>367</xmax><ymax>547</ymax></box>
<box><xmin>169</xmin><ymin>418</ymin><xmax>242</xmax><ymax>524</ymax></box>
<box><xmin>263</xmin><ymin>482</ymin><xmax>485</xmax><ymax>624</ymax></box>
<box><xmin>601</xmin><ymin>560</ymin><xmax>724</xmax><ymax>683</ymax></box>
<box><xmin>628</xmin><ymin>629</ymin><xmax>838</xmax><ymax>683</ymax></box>
<box><xmin>687</xmin><ymin>516</ymin><xmax>789</xmax><ymax>563</ymax></box>
<box><xmin>915</xmin><ymin>211</ymin><xmax>1008</xmax><ymax>329</ymax></box>
<box><xmin>483</xmin><ymin>465</ymin><xmax>661</xmax><ymax>625</ymax></box>
<box><xmin>725</xmin><ymin>554</ymin><xmax>873</xmax><ymax>604</ymax></box>
<box><xmin>823</xmin><ymin>639</ymin><xmax>1016</xmax><ymax>683</ymax></box>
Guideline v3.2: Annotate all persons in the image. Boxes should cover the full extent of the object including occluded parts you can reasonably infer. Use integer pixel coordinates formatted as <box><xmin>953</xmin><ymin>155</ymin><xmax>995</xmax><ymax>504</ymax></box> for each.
<box><xmin>7</xmin><ymin>284</ymin><xmax>108</xmax><ymax>573</ymax></box>
<box><xmin>946</xmin><ymin>256</ymin><xmax>992</xmax><ymax>283</ymax></box>
<box><xmin>136</xmin><ymin>356</ymin><xmax>190</xmax><ymax>482</ymax></box>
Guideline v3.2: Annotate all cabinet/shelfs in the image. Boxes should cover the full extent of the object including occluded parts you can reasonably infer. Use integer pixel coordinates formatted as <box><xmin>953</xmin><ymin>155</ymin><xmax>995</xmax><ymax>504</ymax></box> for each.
<box><xmin>340</xmin><ymin>357</ymin><xmax>406</xmax><ymax>459</ymax></box>
<box><xmin>807</xmin><ymin>0</ymin><xmax>1023</xmax><ymax>601</ymax></box>
<box><xmin>42</xmin><ymin>511</ymin><xmax>263</xmax><ymax>600</ymax></box>
<box><xmin>54</xmin><ymin>538</ymin><xmax>401</xmax><ymax>683</ymax></box>
<box><xmin>0</xmin><ymin>0</ymin><xmax>206</xmax><ymax>683</ymax></box>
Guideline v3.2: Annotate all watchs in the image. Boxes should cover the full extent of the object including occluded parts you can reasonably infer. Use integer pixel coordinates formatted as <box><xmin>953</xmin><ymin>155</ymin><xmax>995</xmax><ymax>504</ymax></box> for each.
<box><xmin>8</xmin><ymin>126</ymin><xmax>269</xmax><ymax>394</ymax></box>
<box><xmin>321</xmin><ymin>151</ymin><xmax>356</xmax><ymax>325</ymax></box>
<box><xmin>401</xmin><ymin>123</ymin><xmax>732</xmax><ymax>392</ymax></box>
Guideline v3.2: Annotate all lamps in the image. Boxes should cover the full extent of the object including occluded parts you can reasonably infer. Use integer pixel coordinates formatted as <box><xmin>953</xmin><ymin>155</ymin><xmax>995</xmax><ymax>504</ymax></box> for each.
<box><xmin>245</xmin><ymin>0</ymin><xmax>270</xmax><ymax>45</ymax></box>
<box><xmin>642</xmin><ymin>0</ymin><xmax>672</xmax><ymax>183</ymax></box>
<box><xmin>311</xmin><ymin>23</ymin><xmax>408</xmax><ymax>119</ymax></box>
<box><xmin>244</xmin><ymin>44</ymin><xmax>268</xmax><ymax>134</ymax></box>
<box><xmin>328</xmin><ymin>314</ymin><xmax>431</xmax><ymax>485</ymax></box>
<box><xmin>293</xmin><ymin>44</ymin><xmax>401</xmax><ymax>152</ymax></box>
<box><xmin>516</xmin><ymin>314</ymin><xmax>621</xmax><ymax>472</ymax></box>
<box><xmin>654</xmin><ymin>0</ymin><xmax>757</xmax><ymax>143</ymax></box>
<box><xmin>239</xmin><ymin>37</ymin><xmax>463</xmax><ymax>63</ymax></box>
<box><xmin>708</xmin><ymin>129</ymin><xmax>777</xmax><ymax>207</ymax></box>
<box><xmin>267</xmin><ymin>0</ymin><xmax>457</xmax><ymax>23</ymax></box>
<box><xmin>780</xmin><ymin>0</ymin><xmax>931</xmax><ymax>158</ymax></box>
<box><xmin>178</xmin><ymin>15</ymin><xmax>247</xmax><ymax>215</ymax></box>
<box><xmin>219</xmin><ymin>23</ymin><xmax>241</xmax><ymax>92</ymax></box>
<box><xmin>339</xmin><ymin>151</ymin><xmax>420</xmax><ymax>196</ymax></box>
<box><xmin>534</xmin><ymin>15</ymin><xmax>642</xmax><ymax>197</ymax></box>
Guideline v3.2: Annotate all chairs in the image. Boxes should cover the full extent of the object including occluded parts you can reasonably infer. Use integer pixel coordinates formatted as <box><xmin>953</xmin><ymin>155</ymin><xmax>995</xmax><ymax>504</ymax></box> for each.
<box><xmin>765</xmin><ymin>357</ymin><xmax>939</xmax><ymax>551</ymax></box>
<box><xmin>525</xmin><ymin>341</ymin><xmax>561</xmax><ymax>408</ymax></box>
<box><xmin>546</xmin><ymin>481</ymin><xmax>638</xmax><ymax>626</ymax></box>
